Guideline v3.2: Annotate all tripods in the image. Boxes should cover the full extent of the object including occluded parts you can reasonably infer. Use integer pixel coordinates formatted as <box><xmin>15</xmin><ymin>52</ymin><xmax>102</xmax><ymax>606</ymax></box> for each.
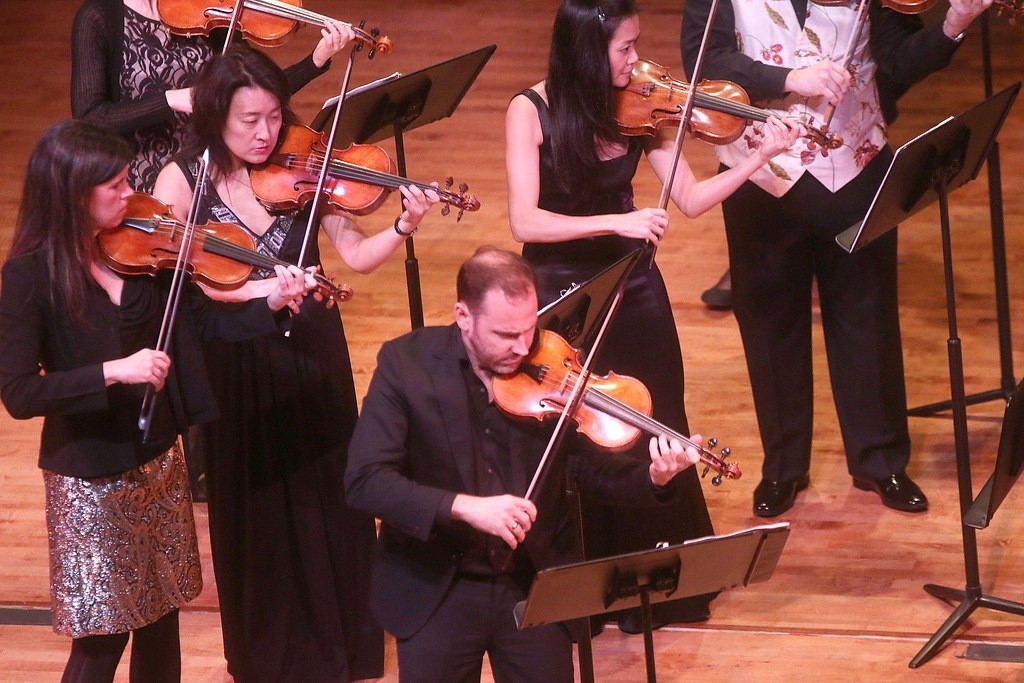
<box><xmin>830</xmin><ymin>7</ymin><xmax>1024</xmax><ymax>669</ymax></box>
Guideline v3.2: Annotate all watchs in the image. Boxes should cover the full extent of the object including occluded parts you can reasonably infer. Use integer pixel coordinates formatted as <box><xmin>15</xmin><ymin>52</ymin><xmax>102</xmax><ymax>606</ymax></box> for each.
<box><xmin>944</xmin><ymin>30</ymin><xmax>967</xmax><ymax>41</ymax></box>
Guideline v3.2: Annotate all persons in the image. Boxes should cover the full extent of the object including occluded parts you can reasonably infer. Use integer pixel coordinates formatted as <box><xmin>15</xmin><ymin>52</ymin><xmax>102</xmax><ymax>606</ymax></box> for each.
<box><xmin>343</xmin><ymin>241</ymin><xmax>703</xmax><ymax>683</ymax></box>
<box><xmin>69</xmin><ymin>0</ymin><xmax>357</xmax><ymax>199</ymax></box>
<box><xmin>157</xmin><ymin>50</ymin><xmax>436</xmax><ymax>683</ymax></box>
<box><xmin>680</xmin><ymin>0</ymin><xmax>1009</xmax><ymax>517</ymax></box>
<box><xmin>0</xmin><ymin>121</ymin><xmax>316</xmax><ymax>683</ymax></box>
<box><xmin>506</xmin><ymin>0</ymin><xmax>807</xmax><ymax>629</ymax></box>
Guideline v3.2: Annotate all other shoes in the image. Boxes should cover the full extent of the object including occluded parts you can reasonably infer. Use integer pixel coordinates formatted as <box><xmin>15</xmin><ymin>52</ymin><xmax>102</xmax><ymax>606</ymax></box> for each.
<box><xmin>701</xmin><ymin>269</ymin><xmax>732</xmax><ymax>307</ymax></box>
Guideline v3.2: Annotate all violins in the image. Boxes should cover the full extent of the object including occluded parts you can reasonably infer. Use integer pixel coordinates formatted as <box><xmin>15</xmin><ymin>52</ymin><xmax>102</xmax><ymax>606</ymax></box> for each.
<box><xmin>248</xmin><ymin>118</ymin><xmax>482</xmax><ymax>227</ymax></box>
<box><xmin>156</xmin><ymin>0</ymin><xmax>395</xmax><ymax>60</ymax></box>
<box><xmin>96</xmin><ymin>188</ymin><xmax>353</xmax><ymax>311</ymax></box>
<box><xmin>490</xmin><ymin>327</ymin><xmax>744</xmax><ymax>491</ymax></box>
<box><xmin>810</xmin><ymin>0</ymin><xmax>1023</xmax><ymax>30</ymax></box>
<box><xmin>608</xmin><ymin>54</ymin><xmax>845</xmax><ymax>157</ymax></box>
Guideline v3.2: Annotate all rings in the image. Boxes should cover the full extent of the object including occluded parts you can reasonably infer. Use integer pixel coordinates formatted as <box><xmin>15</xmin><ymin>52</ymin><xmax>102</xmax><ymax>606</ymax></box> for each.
<box><xmin>289</xmin><ymin>298</ymin><xmax>297</xmax><ymax>305</ymax></box>
<box><xmin>512</xmin><ymin>523</ymin><xmax>518</xmax><ymax>529</ymax></box>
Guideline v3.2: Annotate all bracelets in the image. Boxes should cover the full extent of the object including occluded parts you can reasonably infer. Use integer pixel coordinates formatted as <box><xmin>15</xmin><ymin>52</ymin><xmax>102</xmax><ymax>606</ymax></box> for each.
<box><xmin>395</xmin><ymin>213</ymin><xmax>419</xmax><ymax>239</ymax></box>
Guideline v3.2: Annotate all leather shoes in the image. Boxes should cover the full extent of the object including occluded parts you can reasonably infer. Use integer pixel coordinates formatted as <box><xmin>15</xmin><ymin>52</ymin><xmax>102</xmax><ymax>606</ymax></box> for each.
<box><xmin>753</xmin><ymin>469</ymin><xmax>810</xmax><ymax>517</ymax></box>
<box><xmin>853</xmin><ymin>471</ymin><xmax>928</xmax><ymax>512</ymax></box>
